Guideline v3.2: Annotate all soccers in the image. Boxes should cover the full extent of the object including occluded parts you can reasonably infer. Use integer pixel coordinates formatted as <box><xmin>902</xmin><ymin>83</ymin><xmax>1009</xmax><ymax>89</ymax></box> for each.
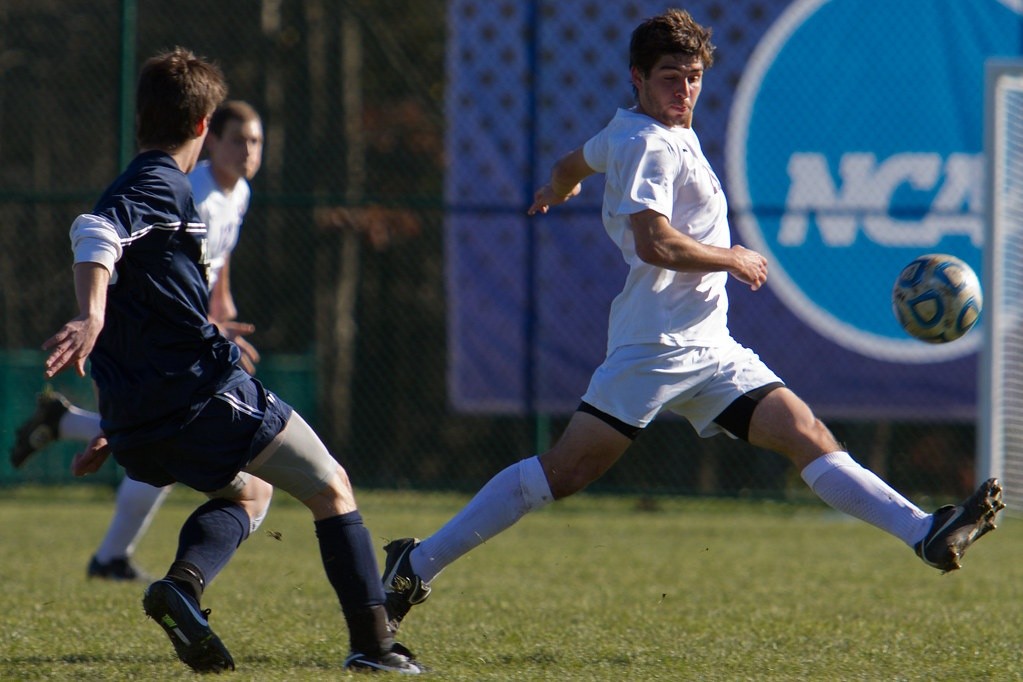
<box><xmin>891</xmin><ymin>253</ymin><xmax>985</xmax><ymax>347</ymax></box>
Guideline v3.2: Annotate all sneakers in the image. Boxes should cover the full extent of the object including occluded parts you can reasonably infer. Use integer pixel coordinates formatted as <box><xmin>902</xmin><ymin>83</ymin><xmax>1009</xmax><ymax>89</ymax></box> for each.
<box><xmin>87</xmin><ymin>556</ymin><xmax>150</xmax><ymax>583</ymax></box>
<box><xmin>381</xmin><ymin>538</ymin><xmax>431</xmax><ymax>636</ymax></box>
<box><xmin>914</xmin><ymin>477</ymin><xmax>1006</xmax><ymax>573</ymax></box>
<box><xmin>10</xmin><ymin>392</ymin><xmax>72</xmax><ymax>468</ymax></box>
<box><xmin>143</xmin><ymin>575</ymin><xmax>235</xmax><ymax>675</ymax></box>
<box><xmin>345</xmin><ymin>641</ymin><xmax>432</xmax><ymax>676</ymax></box>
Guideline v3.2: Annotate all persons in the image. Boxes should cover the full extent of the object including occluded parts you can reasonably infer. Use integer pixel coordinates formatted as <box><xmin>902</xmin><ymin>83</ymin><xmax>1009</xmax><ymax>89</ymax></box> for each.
<box><xmin>10</xmin><ymin>99</ymin><xmax>269</xmax><ymax>582</ymax></box>
<box><xmin>42</xmin><ymin>50</ymin><xmax>426</xmax><ymax>677</ymax></box>
<box><xmin>379</xmin><ymin>10</ymin><xmax>1008</xmax><ymax>630</ymax></box>
